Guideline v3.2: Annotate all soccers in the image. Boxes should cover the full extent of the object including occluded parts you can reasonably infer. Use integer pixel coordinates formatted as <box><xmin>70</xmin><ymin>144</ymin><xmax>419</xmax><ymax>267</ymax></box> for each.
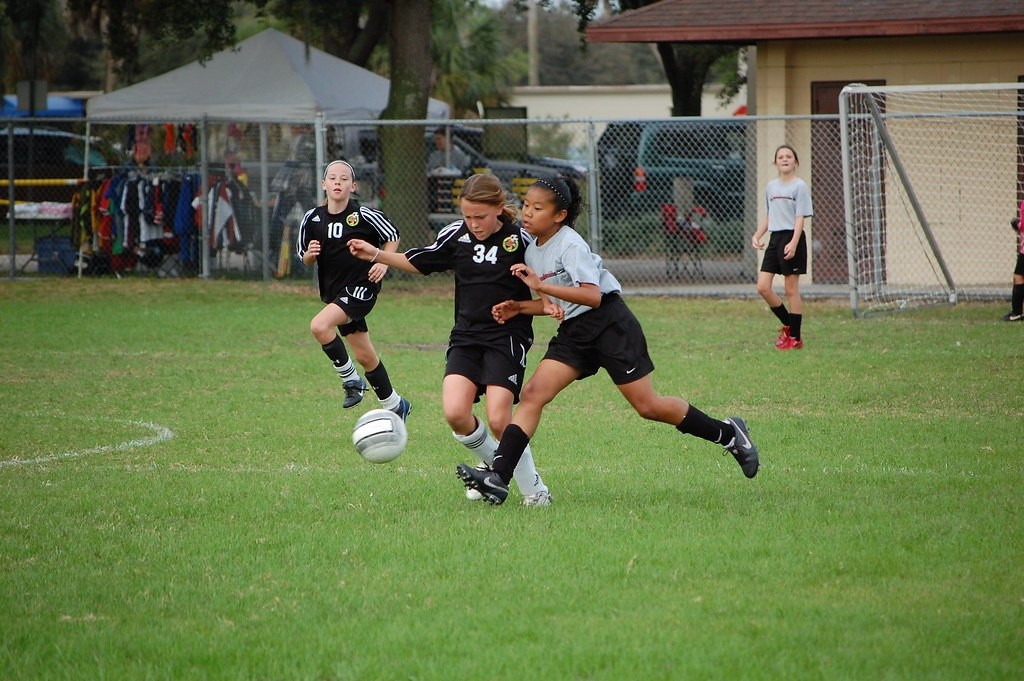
<box><xmin>352</xmin><ymin>408</ymin><xmax>409</xmax><ymax>463</ymax></box>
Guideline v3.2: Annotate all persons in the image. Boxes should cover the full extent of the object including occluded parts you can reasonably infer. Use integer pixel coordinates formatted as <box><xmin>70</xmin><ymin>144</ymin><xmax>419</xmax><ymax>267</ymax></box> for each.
<box><xmin>1001</xmin><ymin>199</ymin><xmax>1024</xmax><ymax>321</ymax></box>
<box><xmin>751</xmin><ymin>144</ymin><xmax>813</xmax><ymax>351</ymax></box>
<box><xmin>457</xmin><ymin>171</ymin><xmax>762</xmax><ymax>505</ymax></box>
<box><xmin>426</xmin><ymin>128</ymin><xmax>469</xmax><ymax>175</ymax></box>
<box><xmin>347</xmin><ymin>172</ymin><xmax>554</xmax><ymax>509</ymax></box>
<box><xmin>297</xmin><ymin>158</ymin><xmax>412</xmax><ymax>424</ymax></box>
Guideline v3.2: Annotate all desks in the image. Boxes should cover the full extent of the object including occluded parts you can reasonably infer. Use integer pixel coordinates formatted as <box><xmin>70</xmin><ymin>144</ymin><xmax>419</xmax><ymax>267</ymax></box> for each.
<box><xmin>5</xmin><ymin>215</ymin><xmax>76</xmax><ymax>274</ymax></box>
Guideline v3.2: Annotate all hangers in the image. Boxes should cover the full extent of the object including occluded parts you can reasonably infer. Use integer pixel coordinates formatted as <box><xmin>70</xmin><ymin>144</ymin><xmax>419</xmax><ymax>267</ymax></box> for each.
<box><xmin>109</xmin><ymin>165</ymin><xmax>204</xmax><ymax>176</ymax></box>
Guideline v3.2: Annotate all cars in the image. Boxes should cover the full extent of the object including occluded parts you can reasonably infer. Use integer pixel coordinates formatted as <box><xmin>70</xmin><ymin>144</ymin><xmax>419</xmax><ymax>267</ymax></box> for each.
<box><xmin>0</xmin><ymin>126</ymin><xmax>138</xmax><ymax>226</ymax></box>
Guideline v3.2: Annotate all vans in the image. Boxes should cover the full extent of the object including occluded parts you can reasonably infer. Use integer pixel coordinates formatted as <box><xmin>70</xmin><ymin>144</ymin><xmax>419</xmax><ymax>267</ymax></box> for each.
<box><xmin>596</xmin><ymin>121</ymin><xmax>748</xmax><ymax>222</ymax></box>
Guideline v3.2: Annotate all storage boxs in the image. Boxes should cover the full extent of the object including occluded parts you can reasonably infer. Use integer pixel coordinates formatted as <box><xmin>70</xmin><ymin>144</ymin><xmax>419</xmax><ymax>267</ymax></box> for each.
<box><xmin>37</xmin><ymin>237</ymin><xmax>77</xmax><ymax>277</ymax></box>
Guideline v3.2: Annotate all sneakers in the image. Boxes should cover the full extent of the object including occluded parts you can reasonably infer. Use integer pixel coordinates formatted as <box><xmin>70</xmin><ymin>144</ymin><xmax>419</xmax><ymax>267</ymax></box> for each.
<box><xmin>391</xmin><ymin>398</ymin><xmax>412</xmax><ymax>423</ymax></box>
<box><xmin>467</xmin><ymin>461</ymin><xmax>493</xmax><ymax>501</ymax></box>
<box><xmin>722</xmin><ymin>417</ymin><xmax>761</xmax><ymax>478</ymax></box>
<box><xmin>775</xmin><ymin>325</ymin><xmax>791</xmax><ymax>345</ymax></box>
<box><xmin>522</xmin><ymin>492</ymin><xmax>553</xmax><ymax>509</ymax></box>
<box><xmin>774</xmin><ymin>335</ymin><xmax>802</xmax><ymax>351</ymax></box>
<box><xmin>1002</xmin><ymin>312</ymin><xmax>1024</xmax><ymax>322</ymax></box>
<box><xmin>456</xmin><ymin>464</ymin><xmax>510</xmax><ymax>505</ymax></box>
<box><xmin>342</xmin><ymin>373</ymin><xmax>369</xmax><ymax>408</ymax></box>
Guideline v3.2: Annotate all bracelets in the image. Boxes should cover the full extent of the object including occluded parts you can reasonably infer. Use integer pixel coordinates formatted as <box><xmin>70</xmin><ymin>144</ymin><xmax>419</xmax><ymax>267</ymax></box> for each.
<box><xmin>370</xmin><ymin>245</ymin><xmax>380</xmax><ymax>262</ymax></box>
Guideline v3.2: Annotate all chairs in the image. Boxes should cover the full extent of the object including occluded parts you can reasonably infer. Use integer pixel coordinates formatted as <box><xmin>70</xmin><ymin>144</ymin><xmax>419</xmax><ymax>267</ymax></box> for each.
<box><xmin>663</xmin><ymin>204</ymin><xmax>711</xmax><ymax>285</ymax></box>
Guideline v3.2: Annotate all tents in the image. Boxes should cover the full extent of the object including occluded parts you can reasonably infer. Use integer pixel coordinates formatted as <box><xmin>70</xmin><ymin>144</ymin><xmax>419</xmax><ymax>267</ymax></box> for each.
<box><xmin>79</xmin><ymin>27</ymin><xmax>452</xmax><ymax>285</ymax></box>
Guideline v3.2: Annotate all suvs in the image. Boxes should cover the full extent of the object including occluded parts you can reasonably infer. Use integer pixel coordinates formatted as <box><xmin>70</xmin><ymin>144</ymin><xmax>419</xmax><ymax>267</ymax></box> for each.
<box><xmin>277</xmin><ymin>122</ymin><xmax>590</xmax><ymax>235</ymax></box>
